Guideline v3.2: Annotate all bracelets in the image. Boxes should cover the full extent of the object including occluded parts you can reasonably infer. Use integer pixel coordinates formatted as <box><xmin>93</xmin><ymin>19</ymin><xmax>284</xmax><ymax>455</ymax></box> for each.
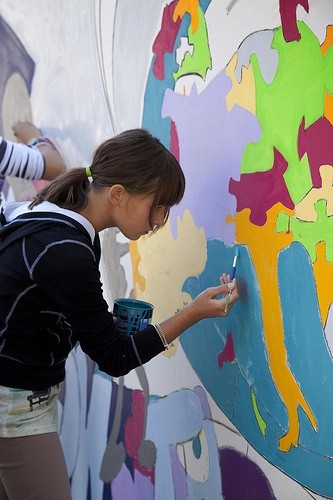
<box><xmin>30</xmin><ymin>137</ymin><xmax>54</xmax><ymax>148</ymax></box>
<box><xmin>153</xmin><ymin>324</ymin><xmax>170</xmax><ymax>351</ymax></box>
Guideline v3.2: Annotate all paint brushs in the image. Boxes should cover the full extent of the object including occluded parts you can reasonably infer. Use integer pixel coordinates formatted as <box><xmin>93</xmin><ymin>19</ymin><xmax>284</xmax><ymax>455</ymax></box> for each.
<box><xmin>223</xmin><ymin>247</ymin><xmax>241</xmax><ymax>313</ymax></box>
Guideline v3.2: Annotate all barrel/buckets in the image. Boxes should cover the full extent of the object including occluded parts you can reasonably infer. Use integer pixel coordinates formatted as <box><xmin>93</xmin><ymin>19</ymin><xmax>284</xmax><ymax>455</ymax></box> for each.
<box><xmin>113</xmin><ymin>298</ymin><xmax>154</xmax><ymax>339</ymax></box>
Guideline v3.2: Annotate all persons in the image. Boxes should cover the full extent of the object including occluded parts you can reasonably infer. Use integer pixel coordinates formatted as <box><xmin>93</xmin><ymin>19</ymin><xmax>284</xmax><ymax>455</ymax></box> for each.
<box><xmin>0</xmin><ymin>121</ymin><xmax>66</xmax><ymax>227</ymax></box>
<box><xmin>0</xmin><ymin>128</ymin><xmax>240</xmax><ymax>500</ymax></box>
